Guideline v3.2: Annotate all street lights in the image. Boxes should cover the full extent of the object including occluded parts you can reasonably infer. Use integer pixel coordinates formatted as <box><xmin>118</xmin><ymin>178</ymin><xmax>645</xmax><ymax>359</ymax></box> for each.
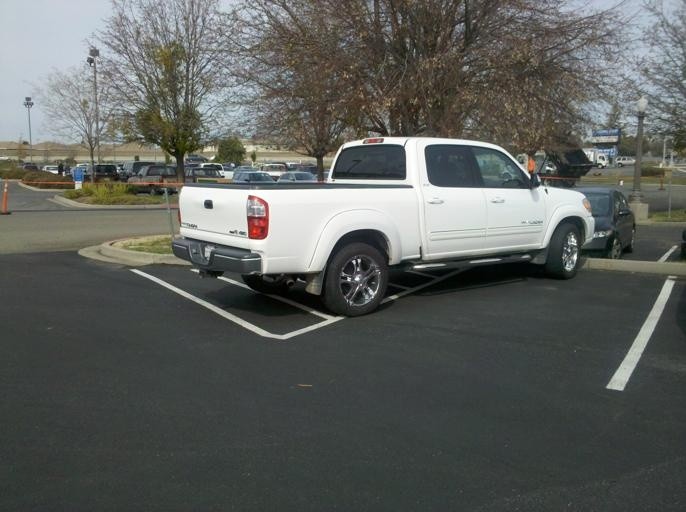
<box><xmin>87</xmin><ymin>46</ymin><xmax>100</xmax><ymax>166</ymax></box>
<box><xmin>22</xmin><ymin>96</ymin><xmax>34</xmax><ymax>161</ymax></box>
<box><xmin>629</xmin><ymin>97</ymin><xmax>649</xmax><ymax>201</ymax></box>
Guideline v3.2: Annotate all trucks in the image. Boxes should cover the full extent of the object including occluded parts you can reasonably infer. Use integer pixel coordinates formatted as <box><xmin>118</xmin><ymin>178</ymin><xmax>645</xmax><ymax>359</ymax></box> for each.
<box><xmin>501</xmin><ymin>131</ymin><xmax>594</xmax><ymax>188</ymax></box>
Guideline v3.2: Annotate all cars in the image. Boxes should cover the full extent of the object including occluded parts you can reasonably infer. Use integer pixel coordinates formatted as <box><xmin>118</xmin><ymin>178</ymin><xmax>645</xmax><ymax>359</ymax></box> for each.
<box><xmin>561</xmin><ymin>186</ymin><xmax>636</xmax><ymax>259</ymax></box>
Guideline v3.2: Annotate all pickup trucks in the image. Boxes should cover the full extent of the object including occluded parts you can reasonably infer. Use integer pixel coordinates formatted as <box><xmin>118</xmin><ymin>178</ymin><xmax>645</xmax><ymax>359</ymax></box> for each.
<box><xmin>170</xmin><ymin>135</ymin><xmax>596</xmax><ymax>319</ymax></box>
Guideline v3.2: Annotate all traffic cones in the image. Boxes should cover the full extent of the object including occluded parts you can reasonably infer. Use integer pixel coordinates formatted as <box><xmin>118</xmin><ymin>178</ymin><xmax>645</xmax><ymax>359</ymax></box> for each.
<box><xmin>0</xmin><ymin>176</ymin><xmax>11</xmax><ymax>215</ymax></box>
